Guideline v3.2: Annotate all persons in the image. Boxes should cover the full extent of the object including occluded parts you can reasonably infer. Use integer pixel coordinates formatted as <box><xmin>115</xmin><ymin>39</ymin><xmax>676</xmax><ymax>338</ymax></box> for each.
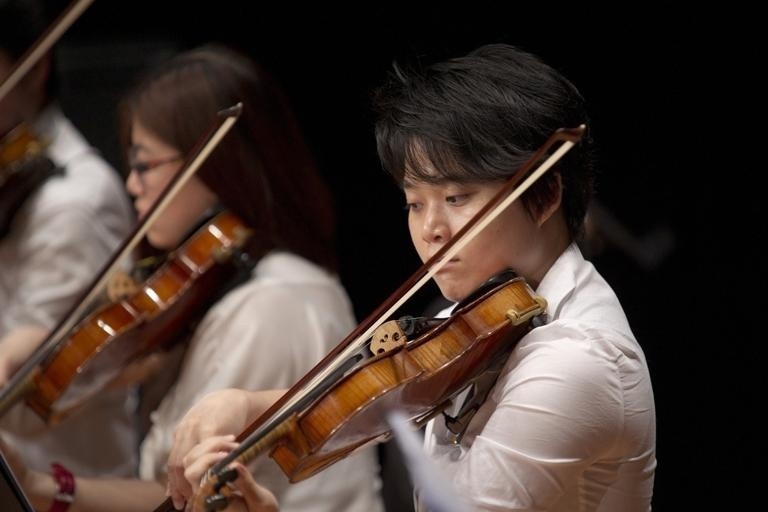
<box><xmin>0</xmin><ymin>0</ymin><xmax>146</xmax><ymax>481</ymax></box>
<box><xmin>1</xmin><ymin>46</ymin><xmax>389</xmax><ymax>511</ymax></box>
<box><xmin>164</xmin><ymin>47</ymin><xmax>658</xmax><ymax>511</ymax></box>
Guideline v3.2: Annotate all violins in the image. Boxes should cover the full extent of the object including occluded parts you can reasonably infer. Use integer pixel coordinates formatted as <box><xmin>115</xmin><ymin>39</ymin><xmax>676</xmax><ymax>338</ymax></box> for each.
<box><xmin>1</xmin><ymin>207</ymin><xmax>257</xmax><ymax>425</ymax></box>
<box><xmin>1</xmin><ymin>121</ymin><xmax>54</xmax><ymax>242</ymax></box>
<box><xmin>198</xmin><ymin>267</ymin><xmax>548</xmax><ymax>510</ymax></box>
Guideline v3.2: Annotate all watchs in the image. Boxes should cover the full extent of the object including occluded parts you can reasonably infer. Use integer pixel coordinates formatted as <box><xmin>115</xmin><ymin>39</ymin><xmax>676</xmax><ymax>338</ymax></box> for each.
<box><xmin>46</xmin><ymin>460</ymin><xmax>74</xmax><ymax>512</ymax></box>
